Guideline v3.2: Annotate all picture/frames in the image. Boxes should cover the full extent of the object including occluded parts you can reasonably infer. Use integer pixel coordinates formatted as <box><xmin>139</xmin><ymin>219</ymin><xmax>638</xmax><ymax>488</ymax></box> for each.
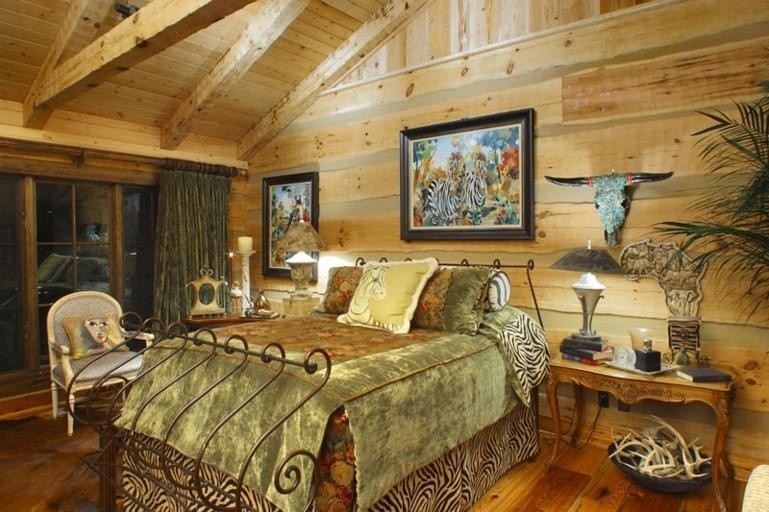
<box><xmin>260</xmin><ymin>171</ymin><xmax>322</xmax><ymax>279</ymax></box>
<box><xmin>398</xmin><ymin>109</ymin><xmax>538</xmax><ymax>244</ymax></box>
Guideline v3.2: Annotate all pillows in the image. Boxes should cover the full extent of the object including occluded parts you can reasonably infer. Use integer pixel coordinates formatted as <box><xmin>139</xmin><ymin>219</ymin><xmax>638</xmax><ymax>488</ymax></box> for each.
<box><xmin>60</xmin><ymin>311</ymin><xmax>132</xmax><ymax>360</ymax></box>
<box><xmin>307</xmin><ymin>256</ymin><xmax>514</xmax><ymax>337</ymax></box>
<box><xmin>37</xmin><ymin>253</ymin><xmax>110</xmax><ymax>287</ymax></box>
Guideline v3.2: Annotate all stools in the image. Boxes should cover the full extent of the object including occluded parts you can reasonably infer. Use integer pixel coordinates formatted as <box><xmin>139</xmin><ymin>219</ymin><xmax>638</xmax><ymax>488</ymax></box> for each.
<box><xmin>739</xmin><ymin>462</ymin><xmax>769</xmax><ymax>512</ymax></box>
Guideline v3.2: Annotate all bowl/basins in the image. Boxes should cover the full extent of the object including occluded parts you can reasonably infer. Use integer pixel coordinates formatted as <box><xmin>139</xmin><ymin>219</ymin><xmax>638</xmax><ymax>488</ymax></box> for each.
<box><xmin>606</xmin><ymin>438</ymin><xmax>715</xmax><ymax>492</ymax></box>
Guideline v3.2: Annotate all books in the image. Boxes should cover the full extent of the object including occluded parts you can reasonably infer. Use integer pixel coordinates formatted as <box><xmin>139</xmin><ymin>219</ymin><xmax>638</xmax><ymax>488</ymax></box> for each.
<box><xmin>675</xmin><ymin>369</ymin><xmax>730</xmax><ymax>383</ymax></box>
<box><xmin>559</xmin><ymin>336</ymin><xmax>612</xmax><ymax>364</ymax></box>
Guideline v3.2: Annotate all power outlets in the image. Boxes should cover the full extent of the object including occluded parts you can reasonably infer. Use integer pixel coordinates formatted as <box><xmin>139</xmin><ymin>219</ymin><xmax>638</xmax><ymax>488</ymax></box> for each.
<box><xmin>597</xmin><ymin>390</ymin><xmax>608</xmax><ymax>408</ymax></box>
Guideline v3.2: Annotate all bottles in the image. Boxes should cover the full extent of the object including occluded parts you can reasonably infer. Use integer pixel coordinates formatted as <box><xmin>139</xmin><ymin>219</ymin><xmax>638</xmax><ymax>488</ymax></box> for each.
<box><xmin>634</xmin><ymin>338</ymin><xmax>662</xmax><ymax>371</ymax></box>
<box><xmin>230</xmin><ymin>281</ymin><xmax>242</xmax><ymax>314</ymax></box>
<box><xmin>254</xmin><ymin>288</ymin><xmax>271</xmax><ymax>313</ymax></box>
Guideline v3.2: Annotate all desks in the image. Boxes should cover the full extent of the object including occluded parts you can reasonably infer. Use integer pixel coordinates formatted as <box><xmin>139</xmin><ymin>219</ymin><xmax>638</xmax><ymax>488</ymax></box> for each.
<box><xmin>542</xmin><ymin>353</ymin><xmax>740</xmax><ymax>512</ymax></box>
<box><xmin>183</xmin><ymin>314</ymin><xmax>278</xmax><ymax>333</ymax></box>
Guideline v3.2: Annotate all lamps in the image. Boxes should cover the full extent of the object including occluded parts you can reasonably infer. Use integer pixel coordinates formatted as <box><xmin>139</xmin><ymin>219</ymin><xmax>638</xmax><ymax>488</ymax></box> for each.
<box><xmin>270</xmin><ymin>222</ymin><xmax>330</xmax><ymax>299</ymax></box>
<box><xmin>545</xmin><ymin>239</ymin><xmax>628</xmax><ymax>340</ymax></box>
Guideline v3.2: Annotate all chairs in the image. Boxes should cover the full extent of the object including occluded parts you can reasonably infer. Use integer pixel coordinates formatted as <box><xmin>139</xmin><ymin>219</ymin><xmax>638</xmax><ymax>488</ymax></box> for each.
<box><xmin>44</xmin><ymin>288</ymin><xmax>156</xmax><ymax>438</ymax></box>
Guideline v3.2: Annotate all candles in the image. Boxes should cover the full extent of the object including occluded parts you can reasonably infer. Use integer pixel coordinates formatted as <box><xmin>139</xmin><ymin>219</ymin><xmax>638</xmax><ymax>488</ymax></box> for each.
<box><xmin>236</xmin><ymin>234</ymin><xmax>254</xmax><ymax>251</ymax></box>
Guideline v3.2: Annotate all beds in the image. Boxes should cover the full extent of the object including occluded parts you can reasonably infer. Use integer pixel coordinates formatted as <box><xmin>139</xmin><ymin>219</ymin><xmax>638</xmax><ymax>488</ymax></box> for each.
<box><xmin>61</xmin><ymin>252</ymin><xmax>555</xmax><ymax>512</ymax></box>
<box><xmin>0</xmin><ymin>280</ymin><xmax>110</xmax><ymax>328</ymax></box>
